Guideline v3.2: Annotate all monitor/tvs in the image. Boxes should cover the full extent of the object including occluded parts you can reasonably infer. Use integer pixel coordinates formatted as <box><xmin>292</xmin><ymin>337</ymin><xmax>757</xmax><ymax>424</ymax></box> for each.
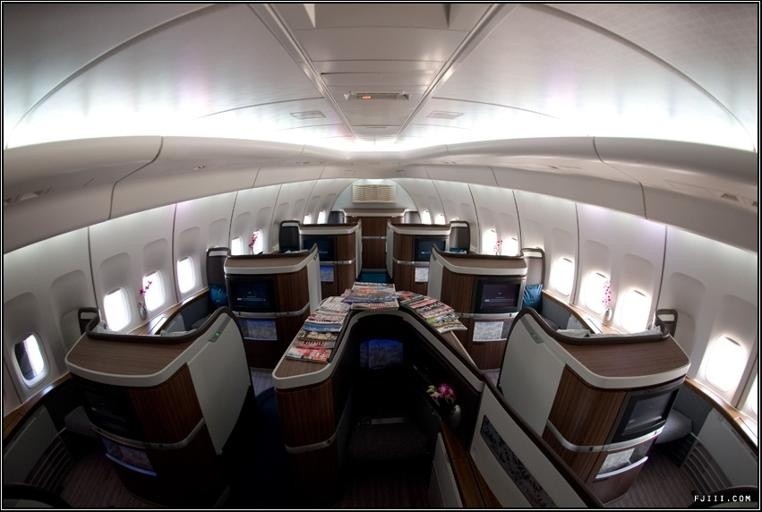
<box><xmin>303</xmin><ymin>235</ymin><xmax>337</xmax><ymax>261</ymax></box>
<box><xmin>469</xmin><ymin>277</ymin><xmax>521</xmax><ymax>312</ymax></box>
<box><xmin>612</xmin><ymin>388</ymin><xmax>679</xmax><ymax>445</ymax></box>
<box><xmin>414</xmin><ymin>235</ymin><xmax>446</xmax><ymax>260</ymax></box>
<box><xmin>72</xmin><ymin>377</ymin><xmax>138</xmax><ymax>440</ymax></box>
<box><xmin>230</xmin><ymin>276</ymin><xmax>281</xmax><ymax>312</ymax></box>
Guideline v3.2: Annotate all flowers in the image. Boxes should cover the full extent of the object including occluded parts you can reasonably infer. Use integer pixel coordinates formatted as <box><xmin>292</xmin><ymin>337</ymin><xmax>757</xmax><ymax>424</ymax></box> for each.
<box><xmin>425</xmin><ymin>382</ymin><xmax>459</xmax><ymax>419</ymax></box>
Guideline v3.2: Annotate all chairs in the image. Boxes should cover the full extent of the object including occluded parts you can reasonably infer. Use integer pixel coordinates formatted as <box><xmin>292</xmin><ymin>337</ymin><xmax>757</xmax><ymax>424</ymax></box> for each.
<box><xmin>65</xmin><ymin>301</ymin><xmax>251</xmax><ymax>490</ymax></box>
<box><xmin>207</xmin><ymin>243</ymin><xmax>325</xmax><ymax>369</ymax></box>
<box><xmin>386</xmin><ymin>219</ymin><xmax>471</xmax><ymax>294</ymax></box>
<box><xmin>473</xmin><ymin>304</ymin><xmax>695</xmax><ymax>504</ymax></box>
<box><xmin>427</xmin><ymin>243</ymin><xmax>545</xmax><ymax>370</ymax></box>
<box><xmin>280</xmin><ymin>219</ymin><xmax>364</xmax><ymax>298</ymax></box>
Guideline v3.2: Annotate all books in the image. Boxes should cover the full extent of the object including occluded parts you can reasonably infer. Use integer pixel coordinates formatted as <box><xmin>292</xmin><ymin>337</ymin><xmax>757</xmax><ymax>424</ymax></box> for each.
<box><xmin>287</xmin><ymin>279</ymin><xmax>467</xmax><ymax>363</ymax></box>
<box><xmin>472</xmin><ymin>321</ymin><xmax>505</xmax><ymax>342</ymax></box>
